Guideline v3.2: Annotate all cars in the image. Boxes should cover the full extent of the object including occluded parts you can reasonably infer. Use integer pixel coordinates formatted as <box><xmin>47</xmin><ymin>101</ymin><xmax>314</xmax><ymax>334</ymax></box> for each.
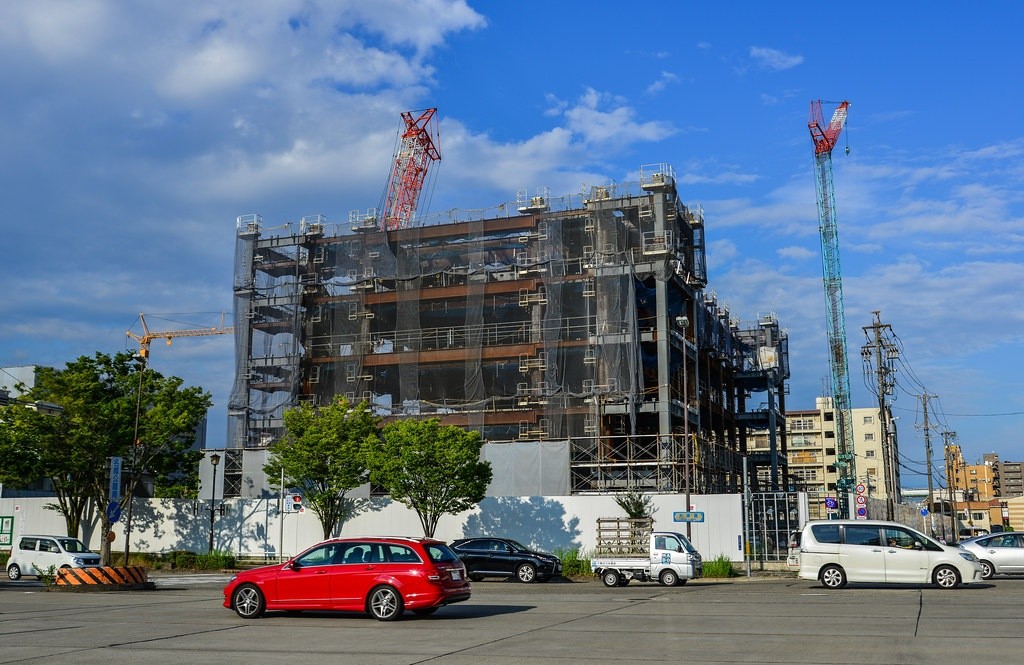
<box><xmin>222</xmin><ymin>536</ymin><xmax>472</xmax><ymax>621</ymax></box>
<box><xmin>6</xmin><ymin>534</ymin><xmax>101</xmax><ymax>581</ymax></box>
<box><xmin>435</xmin><ymin>536</ymin><xmax>563</xmax><ymax>584</ymax></box>
<box><xmin>956</xmin><ymin>531</ymin><xmax>1024</xmax><ymax>580</ymax></box>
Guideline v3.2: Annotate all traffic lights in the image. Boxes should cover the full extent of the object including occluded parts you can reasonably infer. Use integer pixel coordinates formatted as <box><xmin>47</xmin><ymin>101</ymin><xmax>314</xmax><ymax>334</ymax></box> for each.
<box><xmin>291</xmin><ymin>495</ymin><xmax>302</xmax><ymax>511</ymax></box>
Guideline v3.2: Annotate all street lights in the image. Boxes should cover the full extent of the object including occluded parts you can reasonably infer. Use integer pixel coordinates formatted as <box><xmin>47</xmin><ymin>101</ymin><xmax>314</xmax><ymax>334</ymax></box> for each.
<box><xmin>675</xmin><ymin>316</ymin><xmax>691</xmax><ymax>543</ymax></box>
<box><xmin>123</xmin><ymin>353</ymin><xmax>148</xmax><ymax>567</ymax></box>
<box><xmin>205</xmin><ymin>453</ymin><xmax>224</xmax><ymax>556</ymax></box>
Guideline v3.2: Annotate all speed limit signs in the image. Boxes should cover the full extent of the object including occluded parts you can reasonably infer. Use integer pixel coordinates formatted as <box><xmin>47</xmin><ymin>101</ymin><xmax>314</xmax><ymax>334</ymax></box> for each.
<box><xmin>856</xmin><ymin>484</ymin><xmax>865</xmax><ymax>493</ymax></box>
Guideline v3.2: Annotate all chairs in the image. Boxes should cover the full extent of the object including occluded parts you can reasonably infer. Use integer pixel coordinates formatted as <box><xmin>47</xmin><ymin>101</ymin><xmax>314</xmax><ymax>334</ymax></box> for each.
<box><xmin>400</xmin><ymin>554</ymin><xmax>409</xmax><ymax>562</ymax></box>
<box><xmin>39</xmin><ymin>542</ymin><xmax>49</xmax><ymax>552</ymax></box>
<box><xmin>496</xmin><ymin>542</ymin><xmax>506</xmax><ymax>551</ymax></box>
<box><xmin>362</xmin><ymin>551</ymin><xmax>372</xmax><ymax>562</ymax></box>
<box><xmin>390</xmin><ymin>552</ymin><xmax>400</xmax><ymax>562</ymax></box>
<box><xmin>347</xmin><ymin>547</ymin><xmax>364</xmax><ymax>563</ymax></box>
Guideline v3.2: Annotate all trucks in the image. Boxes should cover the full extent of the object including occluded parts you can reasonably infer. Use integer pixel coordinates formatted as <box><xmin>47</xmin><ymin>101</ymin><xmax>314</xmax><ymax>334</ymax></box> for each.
<box><xmin>590</xmin><ymin>531</ymin><xmax>701</xmax><ymax>588</ymax></box>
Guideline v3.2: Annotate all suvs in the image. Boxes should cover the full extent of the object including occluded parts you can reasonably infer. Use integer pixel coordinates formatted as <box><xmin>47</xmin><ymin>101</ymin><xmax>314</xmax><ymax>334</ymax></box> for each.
<box><xmin>797</xmin><ymin>519</ymin><xmax>984</xmax><ymax>589</ymax></box>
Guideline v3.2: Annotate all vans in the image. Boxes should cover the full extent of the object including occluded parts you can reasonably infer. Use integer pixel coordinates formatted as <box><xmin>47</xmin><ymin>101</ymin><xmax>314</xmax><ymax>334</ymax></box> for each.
<box><xmin>960</xmin><ymin>528</ymin><xmax>989</xmax><ymax>540</ymax></box>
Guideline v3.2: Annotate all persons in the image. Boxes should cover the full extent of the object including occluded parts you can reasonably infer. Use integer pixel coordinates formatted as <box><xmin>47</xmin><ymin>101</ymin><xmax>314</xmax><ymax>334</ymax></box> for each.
<box><xmin>890</xmin><ymin>538</ymin><xmax>912</xmax><ymax>548</ymax></box>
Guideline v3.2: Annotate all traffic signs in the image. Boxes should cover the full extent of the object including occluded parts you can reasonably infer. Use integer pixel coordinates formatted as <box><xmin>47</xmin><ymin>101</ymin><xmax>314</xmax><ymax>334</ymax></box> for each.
<box><xmin>673</xmin><ymin>511</ymin><xmax>704</xmax><ymax>522</ymax></box>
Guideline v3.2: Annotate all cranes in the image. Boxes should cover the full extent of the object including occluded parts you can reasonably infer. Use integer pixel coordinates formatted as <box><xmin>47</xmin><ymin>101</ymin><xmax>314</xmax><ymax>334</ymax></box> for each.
<box><xmin>124</xmin><ymin>310</ymin><xmax>260</xmax><ymax>447</ymax></box>
<box><xmin>377</xmin><ymin>106</ymin><xmax>442</xmax><ymax>231</ymax></box>
<box><xmin>807</xmin><ymin>99</ymin><xmax>857</xmax><ymax>518</ymax></box>
